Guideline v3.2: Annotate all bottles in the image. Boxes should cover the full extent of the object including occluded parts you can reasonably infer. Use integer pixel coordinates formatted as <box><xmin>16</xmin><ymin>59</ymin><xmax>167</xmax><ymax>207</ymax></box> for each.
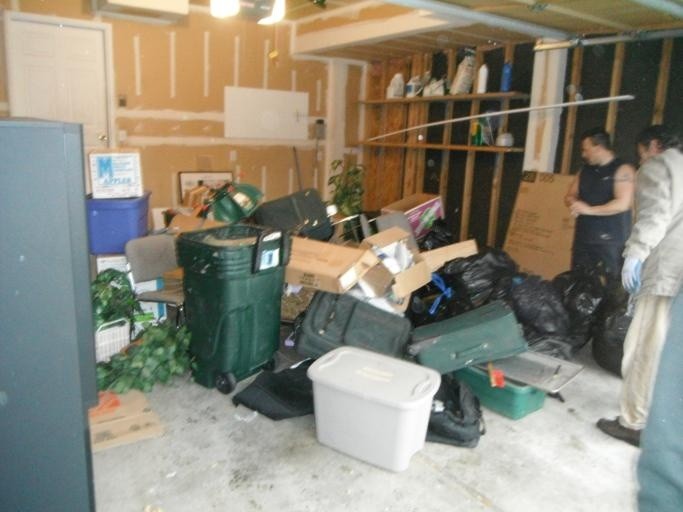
<box><xmin>501</xmin><ymin>60</ymin><xmax>511</xmax><ymax>90</ymax></box>
<box><xmin>390</xmin><ymin>72</ymin><xmax>404</xmax><ymax>97</ymax></box>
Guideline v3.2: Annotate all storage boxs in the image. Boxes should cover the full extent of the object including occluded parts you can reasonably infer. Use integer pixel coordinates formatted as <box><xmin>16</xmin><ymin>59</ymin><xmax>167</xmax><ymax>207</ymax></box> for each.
<box><xmin>304</xmin><ymin>345</ymin><xmax>442</xmax><ymax>475</ymax></box>
<box><xmin>288</xmin><ymin>234</ymin><xmax>375</xmax><ymax>293</ymax></box>
<box><xmin>379</xmin><ymin>191</ymin><xmax>444</xmax><ymax>240</ymax></box>
<box><xmin>86</xmin><ymin>189</ymin><xmax>149</xmax><ymax>254</ymax></box>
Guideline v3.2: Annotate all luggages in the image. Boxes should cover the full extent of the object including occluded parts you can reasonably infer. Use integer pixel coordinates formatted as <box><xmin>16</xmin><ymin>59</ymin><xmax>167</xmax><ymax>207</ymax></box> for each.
<box><xmin>254</xmin><ymin>187</ymin><xmax>333</xmax><ymax>240</ymax></box>
<box><xmin>293</xmin><ymin>290</ymin><xmax>411</xmax><ymax>360</ymax></box>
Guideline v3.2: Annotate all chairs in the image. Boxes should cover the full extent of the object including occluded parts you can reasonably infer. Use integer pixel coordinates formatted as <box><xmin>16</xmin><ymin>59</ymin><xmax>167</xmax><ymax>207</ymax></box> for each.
<box><xmin>123</xmin><ymin>235</ymin><xmax>188</xmax><ymax>339</ymax></box>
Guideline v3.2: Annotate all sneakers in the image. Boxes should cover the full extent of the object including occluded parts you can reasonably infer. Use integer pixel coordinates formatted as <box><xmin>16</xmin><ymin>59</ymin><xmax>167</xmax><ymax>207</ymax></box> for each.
<box><xmin>598</xmin><ymin>417</ymin><xmax>641</xmax><ymax>445</ymax></box>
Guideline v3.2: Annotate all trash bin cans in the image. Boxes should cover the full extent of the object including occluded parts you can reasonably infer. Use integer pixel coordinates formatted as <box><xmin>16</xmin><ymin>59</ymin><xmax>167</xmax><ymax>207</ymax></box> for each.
<box><xmin>176</xmin><ymin>222</ymin><xmax>292</xmax><ymax>395</ymax></box>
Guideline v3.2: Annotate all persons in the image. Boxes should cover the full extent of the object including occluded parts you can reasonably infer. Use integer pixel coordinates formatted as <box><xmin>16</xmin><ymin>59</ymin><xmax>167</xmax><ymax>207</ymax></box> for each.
<box><xmin>597</xmin><ymin>122</ymin><xmax>683</xmax><ymax>446</ymax></box>
<box><xmin>563</xmin><ymin>129</ymin><xmax>637</xmax><ymax>275</ymax></box>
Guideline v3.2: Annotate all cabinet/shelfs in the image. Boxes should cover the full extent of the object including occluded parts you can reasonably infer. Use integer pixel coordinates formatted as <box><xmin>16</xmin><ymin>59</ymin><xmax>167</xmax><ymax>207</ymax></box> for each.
<box><xmin>357</xmin><ymin>36</ymin><xmax>533</xmax><ymax>259</ymax></box>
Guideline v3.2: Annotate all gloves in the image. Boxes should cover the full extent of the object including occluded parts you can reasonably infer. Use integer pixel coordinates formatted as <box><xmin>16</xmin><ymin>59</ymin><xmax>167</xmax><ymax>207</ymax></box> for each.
<box><xmin>620</xmin><ymin>255</ymin><xmax>642</xmax><ymax>291</ymax></box>
